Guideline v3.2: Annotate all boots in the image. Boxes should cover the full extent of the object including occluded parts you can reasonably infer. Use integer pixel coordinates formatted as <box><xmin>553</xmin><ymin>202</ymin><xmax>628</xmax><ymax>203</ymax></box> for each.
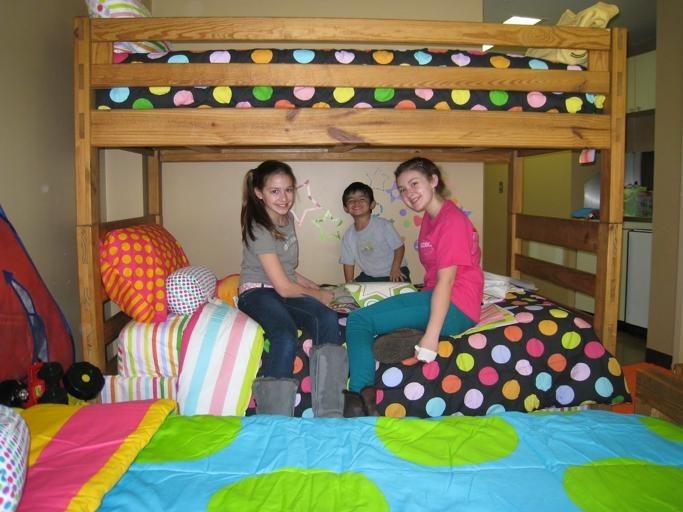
<box><xmin>342</xmin><ymin>386</ymin><xmax>378</xmax><ymax>417</ymax></box>
<box><xmin>308</xmin><ymin>343</ymin><xmax>349</xmax><ymax>417</ymax></box>
<box><xmin>252</xmin><ymin>378</ymin><xmax>300</xmax><ymax>416</ymax></box>
<box><xmin>372</xmin><ymin>328</ymin><xmax>424</xmax><ymax>364</ymax></box>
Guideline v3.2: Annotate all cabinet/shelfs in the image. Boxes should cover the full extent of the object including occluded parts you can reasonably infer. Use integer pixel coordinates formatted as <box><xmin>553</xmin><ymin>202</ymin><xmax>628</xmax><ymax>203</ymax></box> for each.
<box><xmin>574</xmin><ymin>227</ymin><xmax>652</xmax><ymax>340</ymax></box>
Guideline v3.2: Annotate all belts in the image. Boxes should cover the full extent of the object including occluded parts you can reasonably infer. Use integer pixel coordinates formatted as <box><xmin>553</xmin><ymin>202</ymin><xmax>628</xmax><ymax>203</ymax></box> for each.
<box><xmin>238</xmin><ymin>282</ymin><xmax>274</xmax><ymax>296</ymax></box>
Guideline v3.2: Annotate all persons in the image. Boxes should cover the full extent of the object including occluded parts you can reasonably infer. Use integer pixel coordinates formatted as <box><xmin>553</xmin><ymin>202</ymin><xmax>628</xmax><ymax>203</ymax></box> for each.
<box><xmin>337</xmin><ymin>182</ymin><xmax>411</xmax><ymax>282</ymax></box>
<box><xmin>236</xmin><ymin>158</ymin><xmax>349</xmax><ymax>418</ymax></box>
<box><xmin>343</xmin><ymin>154</ymin><xmax>484</xmax><ymax>417</ymax></box>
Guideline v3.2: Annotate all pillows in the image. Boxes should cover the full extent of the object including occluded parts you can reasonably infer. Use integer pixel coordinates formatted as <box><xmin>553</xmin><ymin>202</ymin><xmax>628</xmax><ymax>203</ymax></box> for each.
<box><xmin>1</xmin><ymin>401</ymin><xmax>33</xmax><ymax>511</ymax></box>
<box><xmin>164</xmin><ymin>262</ymin><xmax>218</xmax><ymax>315</ymax></box>
<box><xmin>97</xmin><ymin>222</ymin><xmax>190</xmax><ymax>325</ymax></box>
<box><xmin>84</xmin><ymin>1</ymin><xmax>174</xmax><ymax>64</ymax></box>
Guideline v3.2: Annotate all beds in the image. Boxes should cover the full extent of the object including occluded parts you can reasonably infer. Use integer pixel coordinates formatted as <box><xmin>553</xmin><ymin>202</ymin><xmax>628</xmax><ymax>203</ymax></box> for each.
<box><xmin>1</xmin><ymin>368</ymin><xmax>683</xmax><ymax>512</ymax></box>
<box><xmin>68</xmin><ymin>13</ymin><xmax>630</xmax><ymax>397</ymax></box>
